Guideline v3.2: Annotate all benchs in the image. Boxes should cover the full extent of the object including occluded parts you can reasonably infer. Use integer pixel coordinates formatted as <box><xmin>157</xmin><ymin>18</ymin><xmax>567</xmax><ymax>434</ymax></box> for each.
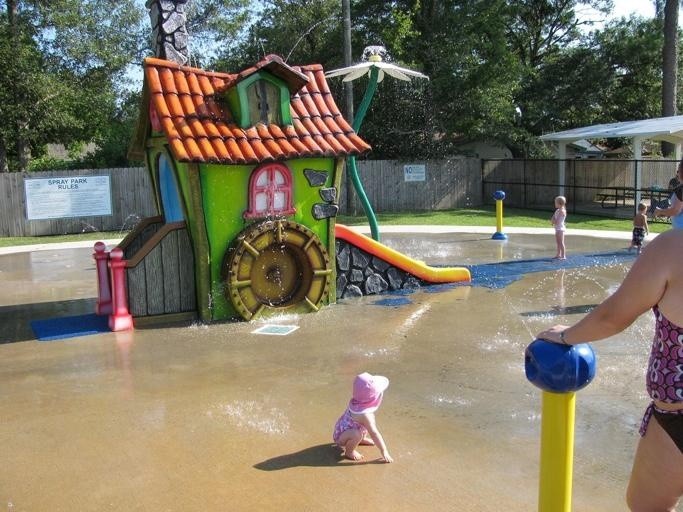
<box><xmin>624</xmin><ymin>192</ymin><xmax>669</xmax><ymax>202</ymax></box>
<box><xmin>594</xmin><ymin>193</ymin><xmax>655</xmax><ymax>208</ymax></box>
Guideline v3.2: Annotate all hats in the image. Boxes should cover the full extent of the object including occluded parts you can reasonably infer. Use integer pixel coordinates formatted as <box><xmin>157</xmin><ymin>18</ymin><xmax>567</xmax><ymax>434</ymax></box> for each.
<box><xmin>348</xmin><ymin>372</ymin><xmax>388</xmax><ymax>414</ymax></box>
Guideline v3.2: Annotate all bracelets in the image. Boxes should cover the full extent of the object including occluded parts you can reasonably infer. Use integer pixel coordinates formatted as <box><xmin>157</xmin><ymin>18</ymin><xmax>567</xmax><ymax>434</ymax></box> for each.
<box><xmin>559</xmin><ymin>329</ymin><xmax>573</xmax><ymax>346</ymax></box>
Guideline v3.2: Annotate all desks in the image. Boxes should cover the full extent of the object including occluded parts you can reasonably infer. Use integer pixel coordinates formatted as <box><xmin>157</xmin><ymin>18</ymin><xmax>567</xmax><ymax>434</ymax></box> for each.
<box><xmin>613</xmin><ymin>185</ymin><xmax>673</xmax><ymax>209</ymax></box>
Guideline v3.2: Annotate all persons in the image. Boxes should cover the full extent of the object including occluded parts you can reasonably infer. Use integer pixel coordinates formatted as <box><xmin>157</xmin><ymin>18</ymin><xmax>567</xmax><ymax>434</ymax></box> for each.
<box><xmin>653</xmin><ymin>157</ymin><xmax>683</xmax><ymax>229</ymax></box>
<box><xmin>551</xmin><ymin>196</ymin><xmax>568</xmax><ymax>260</ymax></box>
<box><xmin>535</xmin><ymin>230</ymin><xmax>683</xmax><ymax>512</ymax></box>
<box><xmin>628</xmin><ymin>202</ymin><xmax>649</xmax><ymax>254</ymax></box>
<box><xmin>668</xmin><ymin>171</ymin><xmax>681</xmax><ymax>198</ymax></box>
<box><xmin>333</xmin><ymin>371</ymin><xmax>394</xmax><ymax>465</ymax></box>
